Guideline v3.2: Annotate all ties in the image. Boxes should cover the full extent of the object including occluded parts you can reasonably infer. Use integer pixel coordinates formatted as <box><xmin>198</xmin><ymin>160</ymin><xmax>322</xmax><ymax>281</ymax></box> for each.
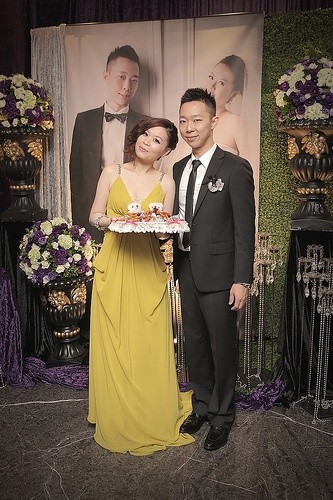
<box><xmin>182</xmin><ymin>160</ymin><xmax>202</xmax><ymax>250</ymax></box>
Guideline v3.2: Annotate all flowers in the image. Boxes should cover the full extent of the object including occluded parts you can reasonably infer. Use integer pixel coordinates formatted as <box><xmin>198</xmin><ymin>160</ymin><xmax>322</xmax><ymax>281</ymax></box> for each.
<box><xmin>0</xmin><ymin>73</ymin><xmax>56</xmax><ymax>128</ymax></box>
<box><xmin>204</xmin><ymin>175</ymin><xmax>223</xmax><ymax>192</ymax></box>
<box><xmin>273</xmin><ymin>56</ymin><xmax>333</xmax><ymax>123</ymax></box>
<box><xmin>19</xmin><ymin>217</ymin><xmax>98</xmax><ymax>287</ymax></box>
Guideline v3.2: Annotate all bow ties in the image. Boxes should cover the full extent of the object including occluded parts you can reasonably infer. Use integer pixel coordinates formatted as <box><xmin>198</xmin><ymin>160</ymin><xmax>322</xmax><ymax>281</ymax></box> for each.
<box><xmin>104</xmin><ymin>112</ymin><xmax>127</xmax><ymax>124</ymax></box>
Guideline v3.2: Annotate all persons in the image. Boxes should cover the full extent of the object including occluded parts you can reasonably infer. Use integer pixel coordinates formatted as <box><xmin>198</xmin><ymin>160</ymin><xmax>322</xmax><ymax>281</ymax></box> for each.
<box><xmin>158</xmin><ymin>89</ymin><xmax>256</xmax><ymax>451</ymax></box>
<box><xmin>69</xmin><ymin>45</ymin><xmax>252</xmax><ymax>252</ymax></box>
<box><xmin>89</xmin><ymin>117</ymin><xmax>179</xmax><ymax>441</ymax></box>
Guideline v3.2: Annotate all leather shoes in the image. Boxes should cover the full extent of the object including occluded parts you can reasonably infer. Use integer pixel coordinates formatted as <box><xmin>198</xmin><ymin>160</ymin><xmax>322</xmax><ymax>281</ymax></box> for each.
<box><xmin>203</xmin><ymin>424</ymin><xmax>231</xmax><ymax>452</ymax></box>
<box><xmin>178</xmin><ymin>411</ymin><xmax>208</xmax><ymax>435</ymax></box>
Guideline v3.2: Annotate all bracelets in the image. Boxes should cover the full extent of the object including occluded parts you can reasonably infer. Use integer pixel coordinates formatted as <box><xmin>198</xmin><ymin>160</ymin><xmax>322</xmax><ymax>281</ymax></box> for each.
<box><xmin>240</xmin><ymin>283</ymin><xmax>251</xmax><ymax>289</ymax></box>
<box><xmin>95</xmin><ymin>215</ymin><xmax>109</xmax><ymax>231</ymax></box>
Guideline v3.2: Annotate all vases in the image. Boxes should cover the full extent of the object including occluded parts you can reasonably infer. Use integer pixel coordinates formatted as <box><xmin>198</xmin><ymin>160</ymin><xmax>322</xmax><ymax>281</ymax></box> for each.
<box><xmin>277</xmin><ymin>119</ymin><xmax>333</xmax><ymax>219</ymax></box>
<box><xmin>0</xmin><ymin>128</ymin><xmax>54</xmax><ymax>221</ymax></box>
<box><xmin>34</xmin><ymin>276</ymin><xmax>88</xmax><ymax>362</ymax></box>
